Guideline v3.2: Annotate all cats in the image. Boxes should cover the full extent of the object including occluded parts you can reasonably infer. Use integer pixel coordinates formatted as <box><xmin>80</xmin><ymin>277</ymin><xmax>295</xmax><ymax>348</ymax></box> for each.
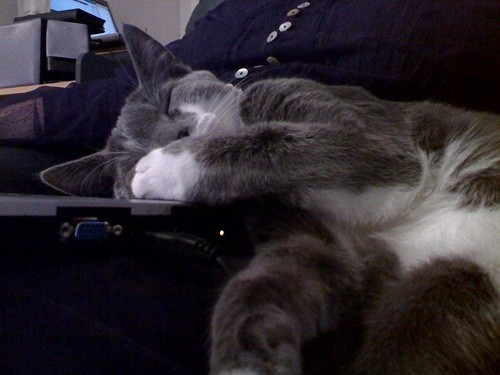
<box><xmin>38</xmin><ymin>22</ymin><xmax>500</xmax><ymax>375</ymax></box>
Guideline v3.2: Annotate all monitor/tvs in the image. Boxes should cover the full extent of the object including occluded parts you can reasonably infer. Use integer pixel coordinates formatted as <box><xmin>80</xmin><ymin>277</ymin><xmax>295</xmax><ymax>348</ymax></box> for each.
<box><xmin>45</xmin><ymin>0</ymin><xmax>120</xmax><ymax>40</ymax></box>
